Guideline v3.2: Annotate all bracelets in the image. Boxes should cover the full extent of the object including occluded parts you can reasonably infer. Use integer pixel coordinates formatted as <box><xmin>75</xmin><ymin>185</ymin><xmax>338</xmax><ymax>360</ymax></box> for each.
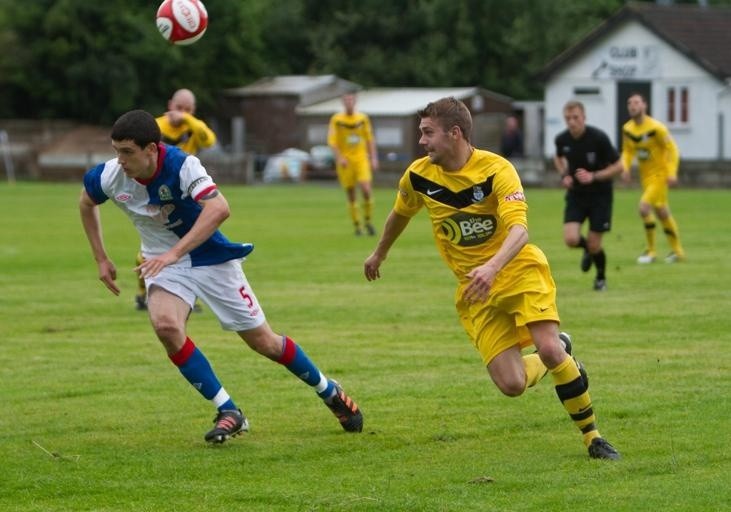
<box><xmin>561</xmin><ymin>171</ymin><xmax>570</xmax><ymax>180</ymax></box>
<box><xmin>591</xmin><ymin>171</ymin><xmax>598</xmax><ymax>183</ymax></box>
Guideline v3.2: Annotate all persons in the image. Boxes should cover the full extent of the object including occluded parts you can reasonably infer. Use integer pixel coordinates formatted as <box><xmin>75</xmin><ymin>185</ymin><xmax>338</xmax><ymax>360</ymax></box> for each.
<box><xmin>328</xmin><ymin>90</ymin><xmax>382</xmax><ymax>236</ymax></box>
<box><xmin>134</xmin><ymin>85</ymin><xmax>215</xmax><ymax>315</ymax></box>
<box><xmin>501</xmin><ymin>112</ymin><xmax>525</xmax><ymax>154</ymax></box>
<box><xmin>365</xmin><ymin>96</ymin><xmax>623</xmax><ymax>460</ymax></box>
<box><xmin>618</xmin><ymin>90</ymin><xmax>685</xmax><ymax>265</ymax></box>
<box><xmin>552</xmin><ymin>102</ymin><xmax>623</xmax><ymax>289</ymax></box>
<box><xmin>78</xmin><ymin>108</ymin><xmax>363</xmax><ymax>443</ymax></box>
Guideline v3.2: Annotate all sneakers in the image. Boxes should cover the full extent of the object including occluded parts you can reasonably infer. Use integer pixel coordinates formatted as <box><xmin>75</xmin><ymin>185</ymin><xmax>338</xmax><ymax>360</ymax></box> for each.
<box><xmin>558</xmin><ymin>332</ymin><xmax>589</xmax><ymax>390</ymax></box>
<box><xmin>205</xmin><ymin>408</ymin><xmax>248</xmax><ymax>443</ymax></box>
<box><xmin>582</xmin><ymin>249</ymin><xmax>592</xmax><ymax>271</ymax></box>
<box><xmin>595</xmin><ymin>278</ymin><xmax>605</xmax><ymax>290</ymax></box>
<box><xmin>588</xmin><ymin>437</ymin><xmax>619</xmax><ymax>460</ymax></box>
<box><xmin>323</xmin><ymin>379</ymin><xmax>363</xmax><ymax>432</ymax></box>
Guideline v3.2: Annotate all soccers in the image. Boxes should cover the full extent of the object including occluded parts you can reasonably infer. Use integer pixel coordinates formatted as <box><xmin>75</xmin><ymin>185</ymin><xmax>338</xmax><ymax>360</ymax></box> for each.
<box><xmin>156</xmin><ymin>0</ymin><xmax>208</xmax><ymax>46</ymax></box>
